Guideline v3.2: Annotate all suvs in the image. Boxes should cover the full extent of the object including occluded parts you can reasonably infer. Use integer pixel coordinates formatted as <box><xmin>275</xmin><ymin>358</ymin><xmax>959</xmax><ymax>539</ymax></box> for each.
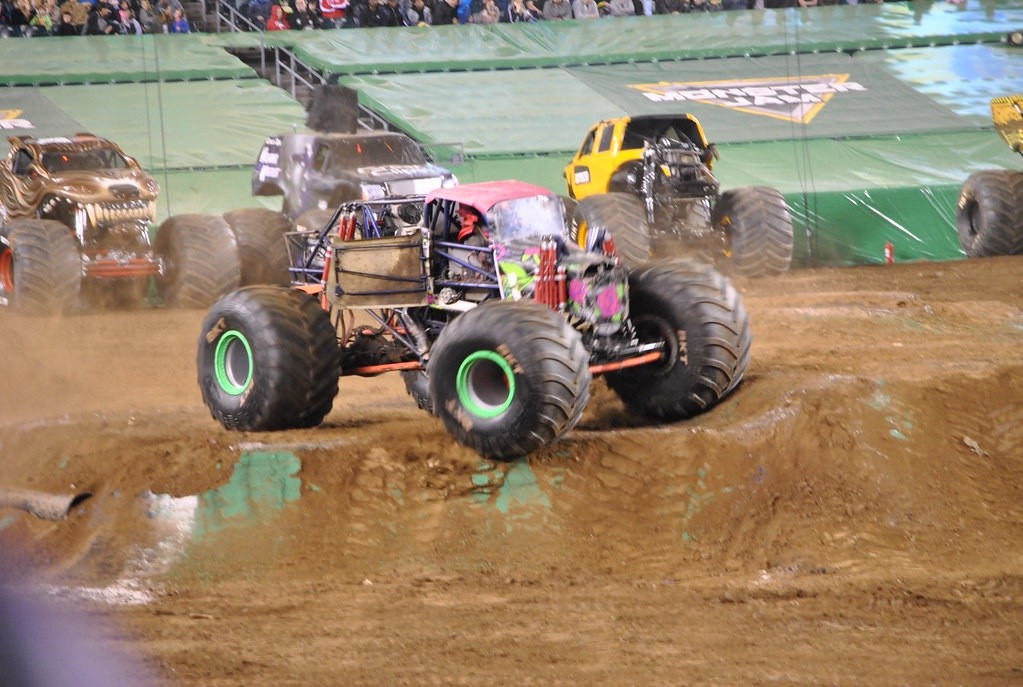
<box><xmin>194</xmin><ymin>178</ymin><xmax>754</xmax><ymax>473</ymax></box>
<box><xmin>227</xmin><ymin>128</ymin><xmax>464</xmax><ymax>285</ymax></box>
<box><xmin>957</xmin><ymin>92</ymin><xmax>1022</xmax><ymax>261</ymax></box>
<box><xmin>559</xmin><ymin>111</ymin><xmax>794</xmax><ymax>285</ymax></box>
<box><xmin>0</xmin><ymin>130</ymin><xmax>241</xmax><ymax>313</ymax></box>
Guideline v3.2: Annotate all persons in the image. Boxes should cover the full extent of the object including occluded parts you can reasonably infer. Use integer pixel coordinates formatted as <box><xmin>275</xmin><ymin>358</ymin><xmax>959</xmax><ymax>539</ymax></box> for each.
<box><xmin>453</xmin><ymin>202</ymin><xmax>488</xmax><ymax>247</ymax></box>
<box><xmin>0</xmin><ymin>0</ymin><xmax>898</xmax><ymax>39</ymax></box>
